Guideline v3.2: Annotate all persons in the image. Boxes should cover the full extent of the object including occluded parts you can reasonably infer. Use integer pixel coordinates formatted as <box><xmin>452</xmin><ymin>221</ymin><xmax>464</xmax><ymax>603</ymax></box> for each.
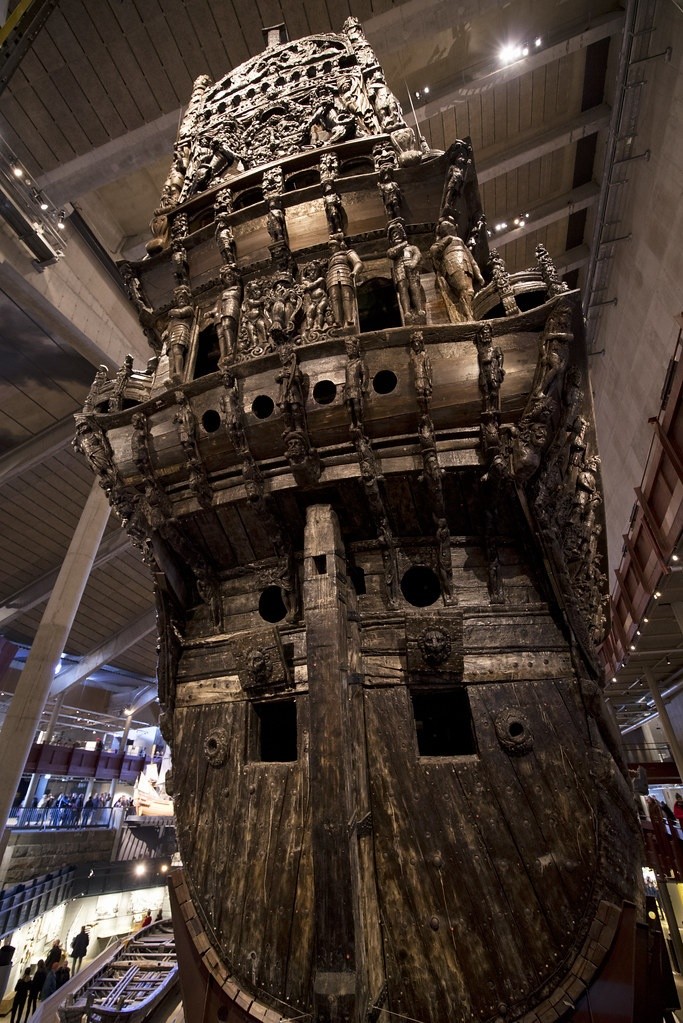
<box><xmin>556</xmin><ymin>418</ymin><xmax>607</xmax><ymax>640</ymax></box>
<box><xmin>10</xmin><ymin>926</ymin><xmax>89</xmax><ymax>1023</ymax></box>
<box><xmin>142</xmin><ymin>910</ymin><xmax>152</xmax><ymax>927</ymax></box>
<box><xmin>139</xmin><ymin>747</ymin><xmax>163</xmax><ymax>758</ymax></box>
<box><xmin>14</xmin><ymin>792</ymin><xmax>136</xmax><ymax>831</ymax></box>
<box><xmin>161</xmin><ymin>285</ymin><xmax>195</xmax><ymax>389</ymax></box>
<box><xmin>85</xmin><ymin>354</ymin><xmax>134</xmax><ymax>410</ymax></box>
<box><xmin>155</xmin><ymin>908</ymin><xmax>162</xmax><ymax>922</ymax></box>
<box><xmin>71</xmin><ymin>371</ymin><xmax>250</xmax><ymax>498</ymax></box>
<box><xmin>168</xmin><ymin>242</ymin><xmax>193</xmax><ymax>287</ymax></box>
<box><xmin>266</xmin><ymin>199</ymin><xmax>287</xmax><ymax>244</ymax></box>
<box><xmin>441</xmin><ymin>157</ymin><xmax>466</xmax><ymax>216</ymax></box>
<box><xmin>95</xmin><ymin>737</ymin><xmax>104</xmax><ymax>752</ymax></box>
<box><xmin>377</xmin><ymin>168</ymin><xmax>404</xmax><ymax>219</ymax></box>
<box><xmin>212</xmin><ymin>213</ymin><xmax>239</xmax><ymax>266</ymax></box>
<box><xmin>320</xmin><ymin>181</ymin><xmax>346</xmax><ymax>233</ymax></box>
<box><xmin>119</xmin><ymin>262</ymin><xmax>154</xmax><ymax>314</ymax></box>
<box><xmin>275</xmin><ymin>306</ymin><xmax>575</xmax><ymax>439</ymax></box>
<box><xmin>384</xmin><ymin>216</ymin><xmax>556</xmax><ymax>322</ymax></box>
<box><xmin>203</xmin><ymin>231</ymin><xmax>363</xmax><ymax>364</ymax></box>
<box><xmin>634</xmin><ymin>791</ymin><xmax>683</xmax><ymax>834</ymax></box>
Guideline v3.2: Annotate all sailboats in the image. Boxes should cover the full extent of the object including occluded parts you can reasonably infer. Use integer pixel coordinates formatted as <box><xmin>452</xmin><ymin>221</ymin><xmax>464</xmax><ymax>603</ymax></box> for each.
<box><xmin>123</xmin><ymin>742</ymin><xmax>178</xmax><ymax>855</ymax></box>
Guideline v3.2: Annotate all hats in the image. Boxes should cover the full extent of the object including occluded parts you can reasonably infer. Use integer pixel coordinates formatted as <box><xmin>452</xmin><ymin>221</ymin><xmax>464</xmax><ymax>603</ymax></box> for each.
<box><xmin>52</xmin><ymin>962</ymin><xmax>59</xmax><ymax>969</ymax></box>
<box><xmin>676</xmin><ymin>793</ymin><xmax>682</xmax><ymax>800</ymax></box>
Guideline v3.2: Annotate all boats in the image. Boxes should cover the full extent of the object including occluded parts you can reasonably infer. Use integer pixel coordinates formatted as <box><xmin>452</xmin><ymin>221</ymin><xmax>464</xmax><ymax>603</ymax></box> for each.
<box><xmin>56</xmin><ymin>915</ymin><xmax>180</xmax><ymax>1023</ymax></box>
